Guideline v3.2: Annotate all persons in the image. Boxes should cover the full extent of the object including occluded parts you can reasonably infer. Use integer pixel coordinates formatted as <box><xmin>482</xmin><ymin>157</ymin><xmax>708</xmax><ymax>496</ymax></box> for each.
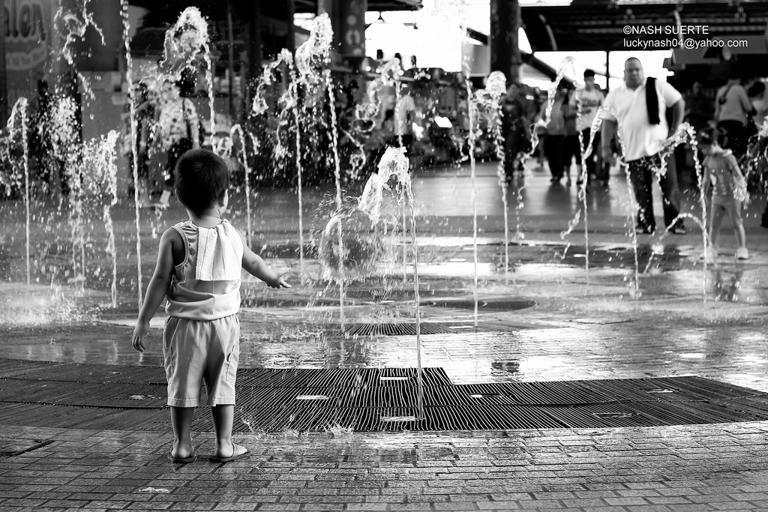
<box><xmin>601</xmin><ymin>57</ymin><xmax>686</xmax><ymax>234</ymax></box>
<box><xmin>697</xmin><ymin>128</ymin><xmax>750</xmax><ymax>260</ymax></box>
<box><xmin>121</xmin><ymin>81</ymin><xmax>156</xmax><ymax>198</ymax></box>
<box><xmin>132</xmin><ymin>149</ymin><xmax>291</xmax><ymax>462</ymax></box>
<box><xmin>714</xmin><ymin>71</ymin><xmax>752</xmax><ymax>158</ymax></box>
<box><xmin>393</xmin><ymin>97</ymin><xmax>417</xmax><ymax>152</ymax></box>
<box><xmin>491</xmin><ymin>70</ymin><xmax>608</xmax><ymax>186</ymax></box>
<box><xmin>152</xmin><ymin>79</ymin><xmax>201</xmax><ymax>210</ymax></box>
<box><xmin>685</xmin><ymin>81</ymin><xmax>713</xmax><ymax>141</ymax></box>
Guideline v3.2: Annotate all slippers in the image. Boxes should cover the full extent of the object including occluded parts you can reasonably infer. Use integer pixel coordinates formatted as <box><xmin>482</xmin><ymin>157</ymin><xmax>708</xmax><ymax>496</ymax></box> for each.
<box><xmin>168</xmin><ymin>445</ymin><xmax>195</xmax><ymax>463</ymax></box>
<box><xmin>208</xmin><ymin>444</ymin><xmax>248</xmax><ymax>462</ymax></box>
